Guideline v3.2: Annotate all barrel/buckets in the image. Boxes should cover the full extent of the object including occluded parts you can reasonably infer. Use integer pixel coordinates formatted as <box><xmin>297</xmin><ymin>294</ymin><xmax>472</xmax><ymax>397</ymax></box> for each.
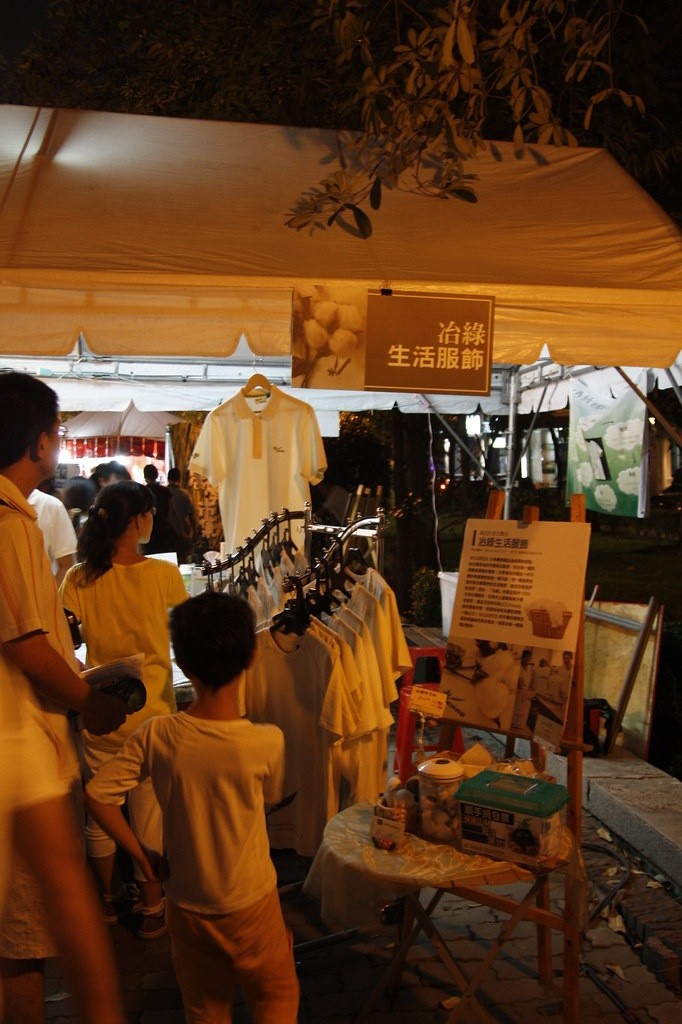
<box><xmin>531</xmin><ymin>609</ymin><xmax>573</xmax><ymax>639</ymax></box>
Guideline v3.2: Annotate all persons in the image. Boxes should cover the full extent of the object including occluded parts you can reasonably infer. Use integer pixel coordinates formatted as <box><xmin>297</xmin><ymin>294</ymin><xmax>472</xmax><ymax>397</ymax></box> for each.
<box><xmin>140</xmin><ymin>463</ymin><xmax>179</xmax><ymax>554</ymax></box>
<box><xmin>1</xmin><ymin>371</ymin><xmax>146</xmax><ymax>1024</ymax></box>
<box><xmin>1</xmin><ymin>661</ymin><xmax>123</xmax><ymax>1024</ymax></box>
<box><xmin>84</xmin><ymin>589</ymin><xmax>300</xmax><ymax>1024</ymax></box>
<box><xmin>57</xmin><ymin>477</ymin><xmax>192</xmax><ymax>941</ymax></box>
<box><xmin>25</xmin><ymin>487</ymin><xmax>79</xmax><ymax>587</ymax></box>
<box><xmin>516</xmin><ymin>649</ymin><xmax>538</xmax><ymax>689</ymax></box>
<box><xmin>165</xmin><ymin>467</ymin><xmax>199</xmax><ymax>564</ymax></box>
<box><xmin>554</xmin><ymin>652</ymin><xmax>575</xmax><ymax>679</ymax></box>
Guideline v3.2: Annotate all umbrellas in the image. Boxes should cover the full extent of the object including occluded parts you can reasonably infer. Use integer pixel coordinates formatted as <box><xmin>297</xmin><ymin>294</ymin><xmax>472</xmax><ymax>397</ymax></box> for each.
<box><xmin>56</xmin><ymin>407</ymin><xmax>186</xmax><ymax>455</ymax></box>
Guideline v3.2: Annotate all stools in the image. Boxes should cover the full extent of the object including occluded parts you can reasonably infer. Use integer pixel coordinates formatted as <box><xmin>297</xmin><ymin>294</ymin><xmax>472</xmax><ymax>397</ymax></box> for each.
<box><xmin>392</xmin><ymin>683</ymin><xmax>465</xmax><ymax>782</ymax></box>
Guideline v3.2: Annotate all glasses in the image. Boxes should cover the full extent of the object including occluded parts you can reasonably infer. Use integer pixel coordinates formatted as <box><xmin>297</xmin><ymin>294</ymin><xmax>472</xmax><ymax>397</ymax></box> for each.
<box><xmin>142</xmin><ymin>507</ymin><xmax>156</xmax><ymax>515</ymax></box>
<box><xmin>42</xmin><ymin>424</ymin><xmax>69</xmax><ymax>439</ymax></box>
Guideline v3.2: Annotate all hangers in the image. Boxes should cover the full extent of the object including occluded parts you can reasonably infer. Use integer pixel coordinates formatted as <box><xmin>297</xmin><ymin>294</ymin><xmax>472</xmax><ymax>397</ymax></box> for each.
<box><xmin>203</xmin><ymin>511</ymin><xmax>310</xmax><ymax>610</ymax></box>
<box><xmin>249</xmin><ymin>528</ymin><xmax>393</xmax><ymax>662</ymax></box>
<box><xmin>208</xmin><ymin>360</ymin><xmax>313</xmax><ymax>418</ymax></box>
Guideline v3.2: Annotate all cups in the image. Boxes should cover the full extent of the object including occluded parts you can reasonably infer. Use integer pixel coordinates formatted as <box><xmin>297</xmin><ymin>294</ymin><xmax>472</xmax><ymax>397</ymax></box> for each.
<box><xmin>405</xmin><ymin>758</ymin><xmax>464</xmax><ymax>841</ymax></box>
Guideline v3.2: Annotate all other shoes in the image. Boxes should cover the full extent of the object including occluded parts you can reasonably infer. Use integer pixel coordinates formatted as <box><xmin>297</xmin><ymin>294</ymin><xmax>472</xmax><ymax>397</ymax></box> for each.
<box><xmin>138</xmin><ymin>899</ymin><xmax>166</xmax><ymax>939</ymax></box>
<box><xmin>131</xmin><ymin>885</ymin><xmax>142</xmax><ymax>915</ymax></box>
<box><xmin>103</xmin><ymin>900</ymin><xmax>117</xmax><ymax>924</ymax></box>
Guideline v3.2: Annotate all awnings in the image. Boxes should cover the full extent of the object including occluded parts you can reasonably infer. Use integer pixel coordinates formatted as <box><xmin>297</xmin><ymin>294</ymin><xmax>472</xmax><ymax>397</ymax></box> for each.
<box><xmin>0</xmin><ymin>103</ymin><xmax>682</xmax><ymax>369</ymax></box>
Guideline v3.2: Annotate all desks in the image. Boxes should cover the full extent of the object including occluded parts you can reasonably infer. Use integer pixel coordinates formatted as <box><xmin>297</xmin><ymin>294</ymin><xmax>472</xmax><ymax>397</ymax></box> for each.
<box><xmin>403</xmin><ymin>627</ymin><xmax>515</xmax><ymax>759</ymax></box>
<box><xmin>302</xmin><ymin>797</ymin><xmax>582</xmax><ymax>1024</ymax></box>
